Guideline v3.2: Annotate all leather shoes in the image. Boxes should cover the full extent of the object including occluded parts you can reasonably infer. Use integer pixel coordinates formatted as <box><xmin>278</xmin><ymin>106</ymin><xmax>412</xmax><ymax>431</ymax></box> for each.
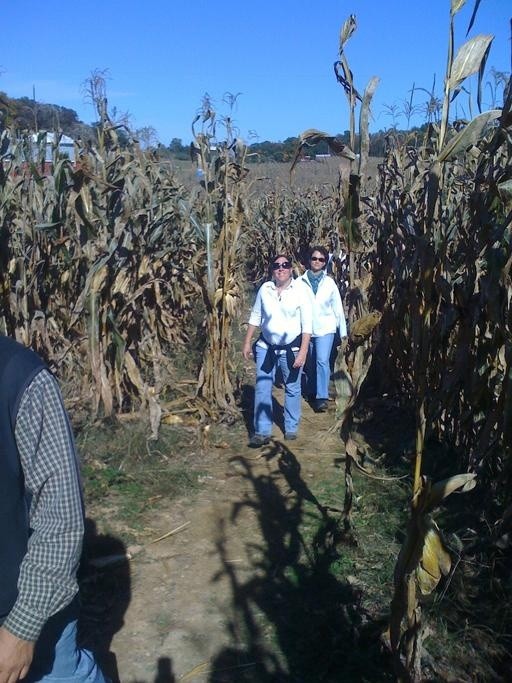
<box><xmin>315</xmin><ymin>399</ymin><xmax>328</xmax><ymax>411</ymax></box>
<box><xmin>284</xmin><ymin>432</ymin><xmax>297</xmax><ymax>439</ymax></box>
<box><xmin>248</xmin><ymin>435</ymin><xmax>270</xmax><ymax>447</ymax></box>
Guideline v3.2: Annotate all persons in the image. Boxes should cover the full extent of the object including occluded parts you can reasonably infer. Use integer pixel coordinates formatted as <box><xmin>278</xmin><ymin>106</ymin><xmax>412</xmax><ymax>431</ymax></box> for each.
<box><xmin>241</xmin><ymin>253</ymin><xmax>314</xmax><ymax>447</ymax></box>
<box><xmin>1</xmin><ymin>335</ymin><xmax>115</xmax><ymax>682</ymax></box>
<box><xmin>293</xmin><ymin>244</ymin><xmax>349</xmax><ymax>411</ymax></box>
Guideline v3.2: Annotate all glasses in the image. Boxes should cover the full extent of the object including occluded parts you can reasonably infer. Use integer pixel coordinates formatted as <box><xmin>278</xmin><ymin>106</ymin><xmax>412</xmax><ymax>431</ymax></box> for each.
<box><xmin>311</xmin><ymin>257</ymin><xmax>326</xmax><ymax>261</ymax></box>
<box><xmin>271</xmin><ymin>261</ymin><xmax>292</xmax><ymax>270</ymax></box>
